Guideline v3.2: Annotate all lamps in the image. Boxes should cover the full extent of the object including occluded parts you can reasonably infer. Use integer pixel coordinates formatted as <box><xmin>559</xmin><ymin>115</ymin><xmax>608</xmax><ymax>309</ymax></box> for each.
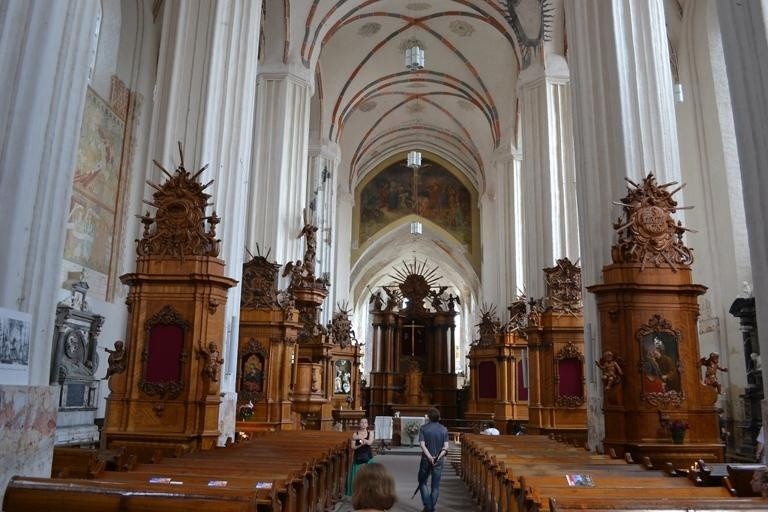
<box><xmin>410</xmin><ymin>221</ymin><xmax>422</xmax><ymax>237</ymax></box>
<box><xmin>405</xmin><ymin>150</ymin><xmax>422</xmax><ymax>169</ymax></box>
<box><xmin>404</xmin><ymin>44</ymin><xmax>425</xmax><ymax>73</ymax></box>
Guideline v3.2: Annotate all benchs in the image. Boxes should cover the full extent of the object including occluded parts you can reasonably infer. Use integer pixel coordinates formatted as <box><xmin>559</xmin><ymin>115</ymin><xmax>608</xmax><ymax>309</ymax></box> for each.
<box><xmin>1</xmin><ymin>431</ymin><xmax>355</xmax><ymax>512</ymax></box>
<box><xmin>459</xmin><ymin>432</ymin><xmax>767</xmax><ymax>509</ymax></box>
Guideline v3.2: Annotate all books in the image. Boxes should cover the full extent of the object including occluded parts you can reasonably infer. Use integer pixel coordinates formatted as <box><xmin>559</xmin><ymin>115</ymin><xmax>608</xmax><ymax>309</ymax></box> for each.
<box><xmin>566</xmin><ymin>473</ymin><xmax>593</xmax><ymax>486</ymax></box>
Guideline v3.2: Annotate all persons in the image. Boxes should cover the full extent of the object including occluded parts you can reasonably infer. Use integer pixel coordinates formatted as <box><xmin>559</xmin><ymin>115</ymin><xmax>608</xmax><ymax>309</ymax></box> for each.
<box><xmin>407</xmin><ymin>277</ymin><xmax>423</xmax><ymax>296</ymax></box>
<box><xmin>344</xmin><ymin>415</ymin><xmax>375</xmax><ymax>496</ymax></box>
<box><xmin>594</xmin><ymin>349</ymin><xmax>623</xmax><ymax>392</ymax></box>
<box><xmin>384</xmin><ymin>289</ymin><xmax>397</xmax><ymax>310</ymax></box>
<box><xmin>295</xmin><ymin>223</ymin><xmax>319</xmax><ymax>248</ymax></box>
<box><xmin>445</xmin><ymin>292</ymin><xmax>456</xmax><ymax>310</ymax></box>
<box><xmin>371</xmin><ymin>292</ymin><xmax>385</xmax><ymax>310</ymax></box>
<box><xmin>428</xmin><ymin>289</ymin><xmax>443</xmax><ymax>306</ymax></box>
<box><xmin>197</xmin><ymin>338</ymin><xmax>224</xmax><ymax>381</ymax></box>
<box><xmin>353</xmin><ymin>461</ymin><xmax>398</xmax><ymax>512</ymax></box>
<box><xmin>290</xmin><ymin>259</ymin><xmax>303</xmax><ymax>289</ymax></box>
<box><xmin>358</xmin><ymin>177</ymin><xmax>462</xmax><ymax>232</ymax></box>
<box><xmin>243</xmin><ymin>363</ymin><xmax>262</xmax><ymax>390</ymax></box>
<box><xmin>481</xmin><ymin>421</ymin><xmax>500</xmax><ymax>435</ymax></box>
<box><xmin>101</xmin><ymin>340</ymin><xmax>126</xmax><ymax>379</ymax></box>
<box><xmin>529</xmin><ymin>305</ymin><xmax>537</xmax><ymax>321</ymax></box>
<box><xmin>754</xmin><ymin>426</ymin><xmax>765</xmax><ymax>462</ymax></box>
<box><xmin>511</xmin><ymin>426</ymin><xmax>526</xmax><ymax>435</ymax></box>
<box><xmin>701</xmin><ymin>351</ymin><xmax>730</xmax><ymax>393</ymax></box>
<box><xmin>416</xmin><ymin>407</ymin><xmax>450</xmax><ymax>510</ymax></box>
<box><xmin>648</xmin><ymin>345</ymin><xmax>678</xmax><ymax>392</ymax></box>
